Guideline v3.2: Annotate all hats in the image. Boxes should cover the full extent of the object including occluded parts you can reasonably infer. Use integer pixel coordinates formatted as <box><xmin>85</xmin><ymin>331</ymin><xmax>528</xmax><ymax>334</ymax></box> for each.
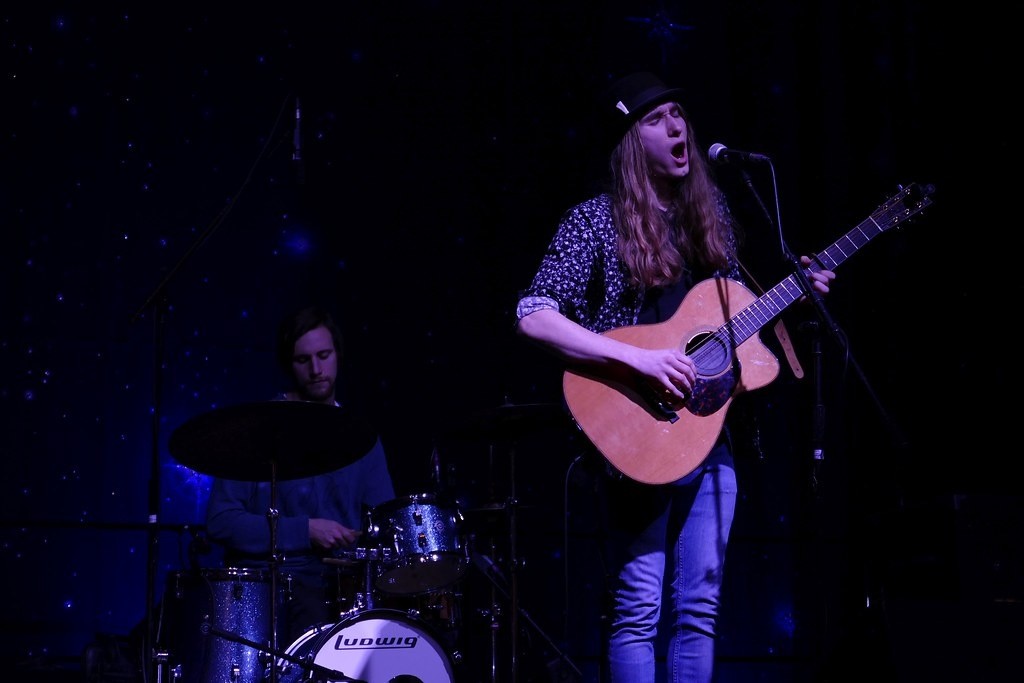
<box><xmin>589</xmin><ymin>59</ymin><xmax>686</xmax><ymax>144</ymax></box>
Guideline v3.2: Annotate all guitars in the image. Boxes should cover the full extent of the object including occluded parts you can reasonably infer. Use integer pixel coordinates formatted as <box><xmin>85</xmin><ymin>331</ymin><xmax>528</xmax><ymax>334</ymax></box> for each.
<box><xmin>557</xmin><ymin>178</ymin><xmax>939</xmax><ymax>489</ymax></box>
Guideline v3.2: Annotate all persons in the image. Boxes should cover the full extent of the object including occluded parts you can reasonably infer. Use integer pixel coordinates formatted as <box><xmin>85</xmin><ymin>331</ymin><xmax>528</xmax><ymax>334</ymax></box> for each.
<box><xmin>204</xmin><ymin>303</ymin><xmax>397</xmax><ymax>567</ymax></box>
<box><xmin>515</xmin><ymin>73</ymin><xmax>836</xmax><ymax>683</ymax></box>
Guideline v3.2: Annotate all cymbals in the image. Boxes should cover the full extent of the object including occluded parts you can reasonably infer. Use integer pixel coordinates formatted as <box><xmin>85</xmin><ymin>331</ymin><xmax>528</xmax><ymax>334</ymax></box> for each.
<box><xmin>478</xmin><ymin>401</ymin><xmax>553</xmax><ymax>412</ymax></box>
<box><xmin>168</xmin><ymin>399</ymin><xmax>377</xmax><ymax>483</ymax></box>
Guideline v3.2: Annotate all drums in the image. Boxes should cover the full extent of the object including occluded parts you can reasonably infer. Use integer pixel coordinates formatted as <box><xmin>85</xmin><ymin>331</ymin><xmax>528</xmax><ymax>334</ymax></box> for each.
<box><xmin>162</xmin><ymin>566</ymin><xmax>280</xmax><ymax>683</ymax></box>
<box><xmin>275</xmin><ymin>607</ymin><xmax>482</xmax><ymax>683</ymax></box>
<box><xmin>363</xmin><ymin>492</ymin><xmax>472</xmax><ymax>598</ymax></box>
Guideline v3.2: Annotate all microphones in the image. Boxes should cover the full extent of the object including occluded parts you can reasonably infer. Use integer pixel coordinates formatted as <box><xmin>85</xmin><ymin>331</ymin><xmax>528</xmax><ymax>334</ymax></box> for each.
<box><xmin>707</xmin><ymin>143</ymin><xmax>770</xmax><ymax>161</ymax></box>
<box><xmin>292</xmin><ymin>95</ymin><xmax>301</xmax><ymax>160</ymax></box>
<box><xmin>433</xmin><ymin>449</ymin><xmax>440</xmax><ymax>485</ymax></box>
<box><xmin>185</xmin><ymin>524</ymin><xmax>213</xmax><ymax>556</ymax></box>
